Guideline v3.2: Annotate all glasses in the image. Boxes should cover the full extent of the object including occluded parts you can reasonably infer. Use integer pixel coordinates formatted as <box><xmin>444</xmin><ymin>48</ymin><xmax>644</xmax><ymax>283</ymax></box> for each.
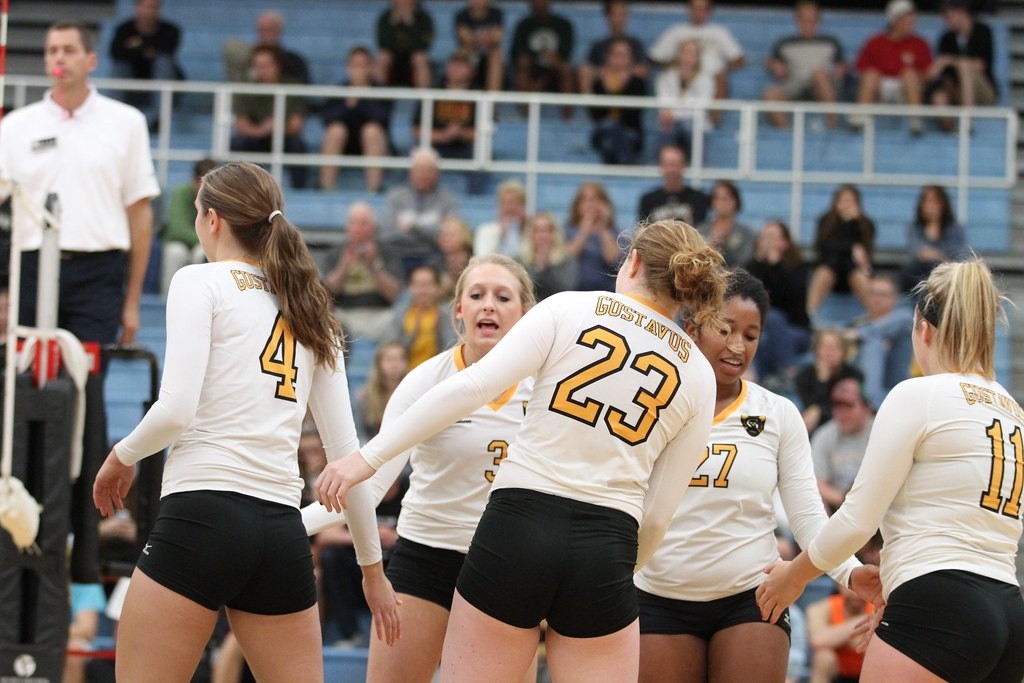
<box><xmin>827</xmin><ymin>397</ymin><xmax>862</xmax><ymax>411</ymax></box>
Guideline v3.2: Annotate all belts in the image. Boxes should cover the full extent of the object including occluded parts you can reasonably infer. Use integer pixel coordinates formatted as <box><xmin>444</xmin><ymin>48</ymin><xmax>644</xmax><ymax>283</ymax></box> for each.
<box><xmin>21</xmin><ymin>250</ymin><xmax>124</xmax><ymax>262</ymax></box>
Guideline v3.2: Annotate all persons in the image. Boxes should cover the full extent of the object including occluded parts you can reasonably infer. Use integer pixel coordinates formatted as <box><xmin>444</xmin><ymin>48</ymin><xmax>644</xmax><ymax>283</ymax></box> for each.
<box><xmin>694</xmin><ymin>181</ymin><xmax>755</xmax><ymax>267</ymax></box>
<box><xmin>0</xmin><ymin>0</ymin><xmax>1024</xmax><ymax>683</ymax></box>
<box><xmin>901</xmin><ymin>187</ymin><xmax>968</xmax><ymax>292</ymax></box>
<box><xmin>92</xmin><ymin>160</ymin><xmax>401</xmax><ymax>683</ymax></box>
<box><xmin>586</xmin><ymin>42</ymin><xmax>648</xmax><ymax>164</ymax></box>
<box><xmin>652</xmin><ymin>39</ymin><xmax>717</xmax><ymax>169</ymax></box>
<box><xmin>472</xmin><ymin>180</ymin><xmax>528</xmax><ymax>262</ymax></box>
<box><xmin>815</xmin><ymin>185</ymin><xmax>875</xmax><ymax>295</ymax></box>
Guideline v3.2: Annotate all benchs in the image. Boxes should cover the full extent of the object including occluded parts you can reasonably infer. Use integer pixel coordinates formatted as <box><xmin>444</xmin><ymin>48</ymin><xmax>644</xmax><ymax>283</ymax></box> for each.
<box><xmin>30</xmin><ymin>0</ymin><xmax>1012</xmax><ymax>637</ymax></box>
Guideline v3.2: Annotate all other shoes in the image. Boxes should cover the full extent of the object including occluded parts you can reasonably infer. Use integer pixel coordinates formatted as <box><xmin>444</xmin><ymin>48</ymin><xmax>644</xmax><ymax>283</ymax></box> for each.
<box><xmin>64</xmin><ymin>626</ymin><xmax>101</xmax><ymax>654</ymax></box>
<box><xmin>907</xmin><ymin>118</ymin><xmax>924</xmax><ymax>136</ymax></box>
<box><xmin>848</xmin><ymin>115</ymin><xmax>868</xmax><ymax>128</ymax></box>
